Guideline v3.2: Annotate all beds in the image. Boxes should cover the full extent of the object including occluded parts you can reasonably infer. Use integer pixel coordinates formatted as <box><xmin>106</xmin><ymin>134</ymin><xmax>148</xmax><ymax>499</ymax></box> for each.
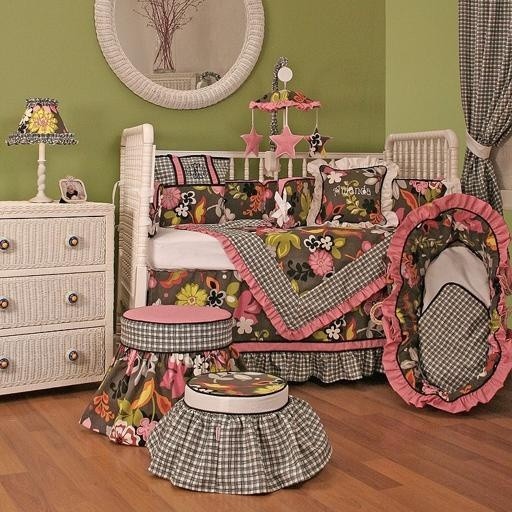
<box><xmin>117</xmin><ymin>123</ymin><xmax>456</xmax><ymax>389</ymax></box>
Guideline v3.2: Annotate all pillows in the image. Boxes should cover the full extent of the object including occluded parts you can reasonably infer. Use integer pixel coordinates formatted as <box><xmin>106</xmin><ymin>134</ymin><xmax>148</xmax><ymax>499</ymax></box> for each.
<box><xmin>156</xmin><ymin>155</ymin><xmax>456</xmax><ymax>233</ymax></box>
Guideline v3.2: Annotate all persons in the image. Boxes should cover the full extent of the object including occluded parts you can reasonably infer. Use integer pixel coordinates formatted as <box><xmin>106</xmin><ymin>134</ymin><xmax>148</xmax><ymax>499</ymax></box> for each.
<box><xmin>66</xmin><ymin>184</ymin><xmax>78</xmax><ymax>200</ymax></box>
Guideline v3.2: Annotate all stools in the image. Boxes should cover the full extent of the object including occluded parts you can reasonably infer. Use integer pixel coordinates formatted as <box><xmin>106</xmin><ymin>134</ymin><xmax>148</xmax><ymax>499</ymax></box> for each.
<box><xmin>78</xmin><ymin>305</ymin><xmax>333</xmax><ymax>497</ymax></box>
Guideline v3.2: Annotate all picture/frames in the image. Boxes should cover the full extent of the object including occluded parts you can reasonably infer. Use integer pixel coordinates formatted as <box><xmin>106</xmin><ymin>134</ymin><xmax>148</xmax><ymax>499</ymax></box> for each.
<box><xmin>91</xmin><ymin>0</ymin><xmax>266</xmax><ymax>112</ymax></box>
<box><xmin>56</xmin><ymin>177</ymin><xmax>89</xmax><ymax>204</ymax></box>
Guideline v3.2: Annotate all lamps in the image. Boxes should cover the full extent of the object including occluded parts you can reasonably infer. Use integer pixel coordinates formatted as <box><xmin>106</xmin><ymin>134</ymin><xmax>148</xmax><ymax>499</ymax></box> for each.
<box><xmin>4</xmin><ymin>97</ymin><xmax>81</xmax><ymax>203</ymax></box>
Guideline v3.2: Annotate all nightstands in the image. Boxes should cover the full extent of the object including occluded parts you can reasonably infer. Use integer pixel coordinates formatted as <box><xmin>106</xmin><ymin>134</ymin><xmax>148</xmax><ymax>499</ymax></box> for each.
<box><xmin>1</xmin><ymin>200</ymin><xmax>117</xmax><ymax>400</ymax></box>
